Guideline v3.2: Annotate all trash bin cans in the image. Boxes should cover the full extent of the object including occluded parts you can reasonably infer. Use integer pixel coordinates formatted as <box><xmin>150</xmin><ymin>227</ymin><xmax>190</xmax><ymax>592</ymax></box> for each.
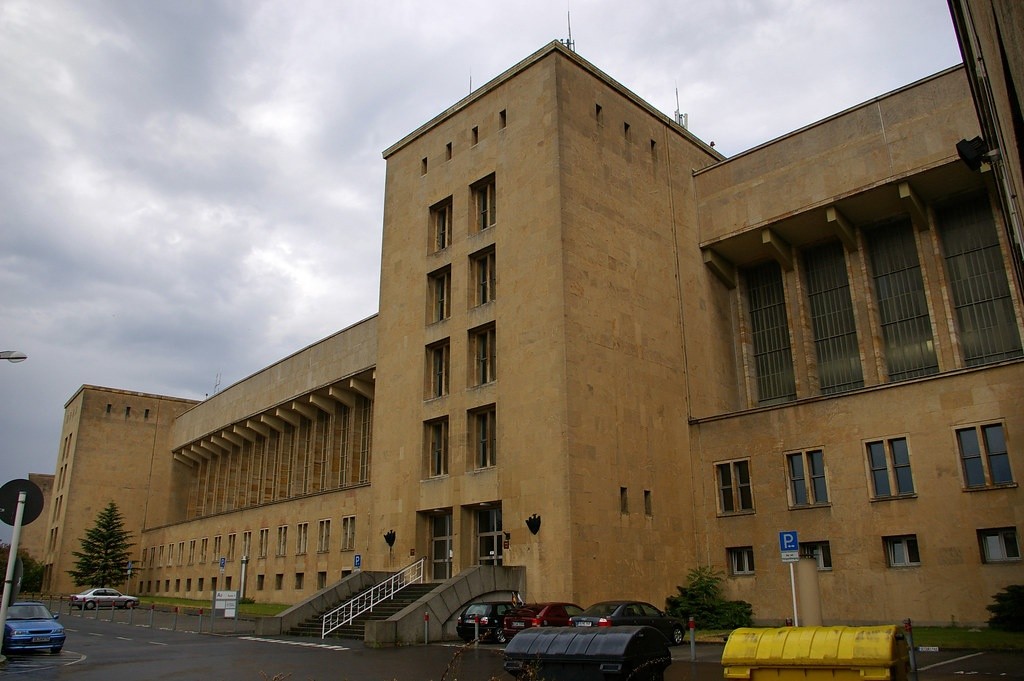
<box><xmin>720</xmin><ymin>625</ymin><xmax>909</xmax><ymax>681</ymax></box>
<box><xmin>503</xmin><ymin>625</ymin><xmax>673</xmax><ymax>681</ymax></box>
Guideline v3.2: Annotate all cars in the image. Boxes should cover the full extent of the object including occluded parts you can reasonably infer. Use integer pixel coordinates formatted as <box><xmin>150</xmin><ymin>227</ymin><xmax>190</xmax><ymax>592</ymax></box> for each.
<box><xmin>3</xmin><ymin>602</ymin><xmax>66</xmax><ymax>656</ymax></box>
<box><xmin>568</xmin><ymin>600</ymin><xmax>686</xmax><ymax>647</ymax></box>
<box><xmin>457</xmin><ymin>600</ymin><xmax>527</xmax><ymax>644</ymax></box>
<box><xmin>501</xmin><ymin>602</ymin><xmax>585</xmax><ymax>645</ymax></box>
<box><xmin>69</xmin><ymin>588</ymin><xmax>140</xmax><ymax>610</ymax></box>
